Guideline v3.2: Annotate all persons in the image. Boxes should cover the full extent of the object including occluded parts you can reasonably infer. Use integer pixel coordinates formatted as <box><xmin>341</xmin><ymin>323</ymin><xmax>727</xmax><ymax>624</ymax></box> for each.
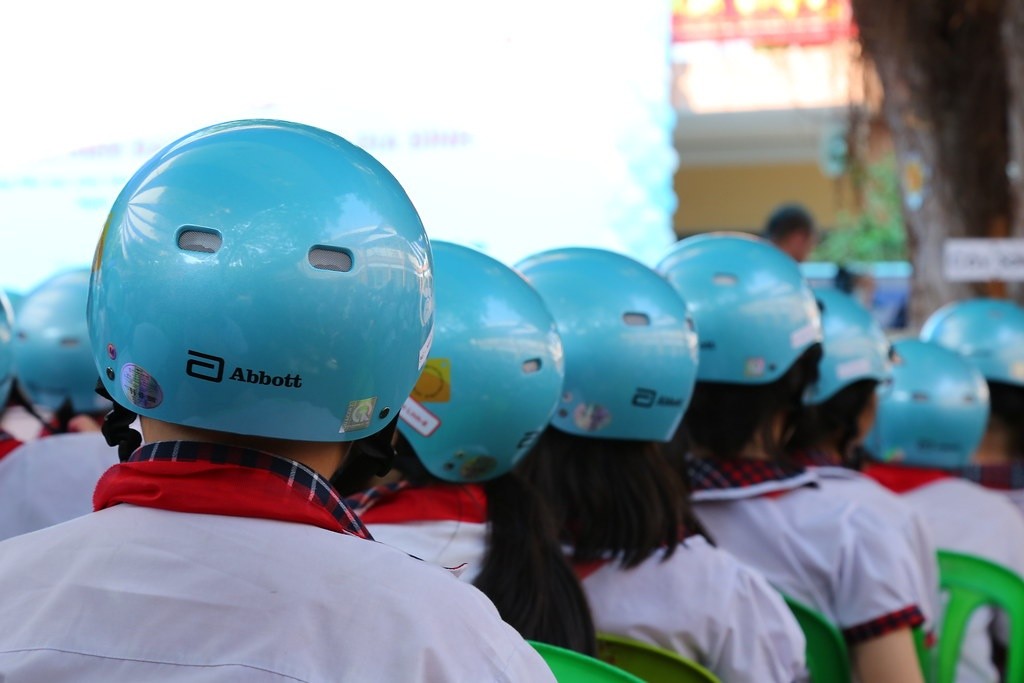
<box><xmin>0</xmin><ymin>117</ymin><xmax>1024</xmax><ymax>683</ymax></box>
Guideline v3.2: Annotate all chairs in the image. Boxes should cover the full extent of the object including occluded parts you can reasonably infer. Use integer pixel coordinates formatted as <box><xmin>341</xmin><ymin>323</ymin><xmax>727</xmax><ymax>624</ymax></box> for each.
<box><xmin>526</xmin><ymin>548</ymin><xmax>1024</xmax><ymax>683</ymax></box>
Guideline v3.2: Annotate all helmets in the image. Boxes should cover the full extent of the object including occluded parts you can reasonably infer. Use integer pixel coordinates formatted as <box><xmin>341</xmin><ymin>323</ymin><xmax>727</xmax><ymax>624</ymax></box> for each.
<box><xmin>514</xmin><ymin>245</ymin><xmax>699</xmax><ymax>442</ymax></box>
<box><xmin>0</xmin><ymin>290</ymin><xmax>19</xmax><ymax>409</ymax></box>
<box><xmin>10</xmin><ymin>266</ymin><xmax>116</xmax><ymax>414</ymax></box>
<box><xmin>85</xmin><ymin>119</ymin><xmax>433</xmax><ymax>441</ymax></box>
<box><xmin>918</xmin><ymin>295</ymin><xmax>1024</xmax><ymax>386</ymax></box>
<box><xmin>800</xmin><ymin>285</ymin><xmax>897</xmax><ymax>406</ymax></box>
<box><xmin>393</xmin><ymin>241</ymin><xmax>565</xmax><ymax>481</ymax></box>
<box><xmin>655</xmin><ymin>230</ymin><xmax>824</xmax><ymax>385</ymax></box>
<box><xmin>861</xmin><ymin>339</ymin><xmax>990</xmax><ymax>469</ymax></box>
